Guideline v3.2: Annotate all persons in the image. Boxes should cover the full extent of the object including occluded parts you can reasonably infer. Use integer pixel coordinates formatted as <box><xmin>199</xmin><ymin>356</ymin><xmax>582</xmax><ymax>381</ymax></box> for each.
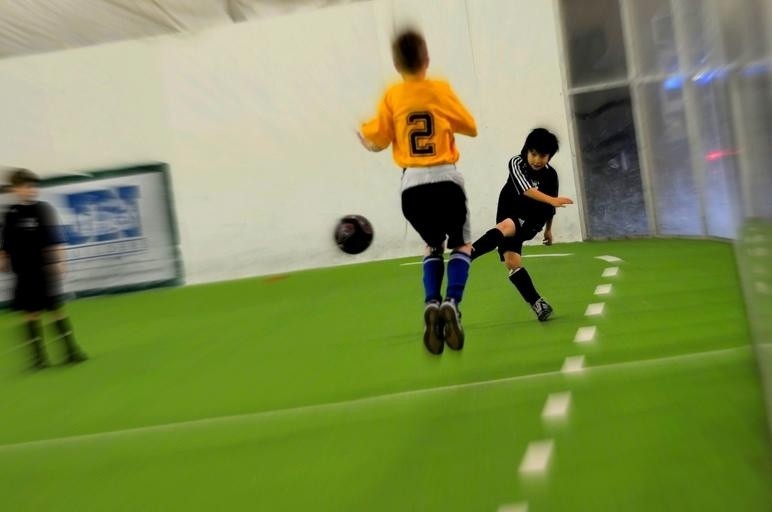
<box><xmin>355</xmin><ymin>30</ymin><xmax>476</xmax><ymax>355</ymax></box>
<box><xmin>472</xmin><ymin>128</ymin><xmax>576</xmax><ymax>321</ymax></box>
<box><xmin>0</xmin><ymin>168</ymin><xmax>92</xmax><ymax>370</ymax></box>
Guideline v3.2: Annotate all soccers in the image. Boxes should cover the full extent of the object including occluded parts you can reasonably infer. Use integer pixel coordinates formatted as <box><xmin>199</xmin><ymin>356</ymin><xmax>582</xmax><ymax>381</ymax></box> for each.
<box><xmin>335</xmin><ymin>215</ymin><xmax>373</xmax><ymax>255</ymax></box>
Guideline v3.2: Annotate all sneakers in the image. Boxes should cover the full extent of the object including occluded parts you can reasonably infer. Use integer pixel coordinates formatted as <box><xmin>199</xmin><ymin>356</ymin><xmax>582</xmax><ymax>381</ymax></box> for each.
<box><xmin>69</xmin><ymin>348</ymin><xmax>87</xmax><ymax>361</ymax></box>
<box><xmin>39</xmin><ymin>355</ymin><xmax>49</xmax><ymax>366</ymax></box>
<box><xmin>531</xmin><ymin>297</ymin><xmax>552</xmax><ymax>320</ymax></box>
<box><xmin>423</xmin><ymin>301</ymin><xmax>464</xmax><ymax>354</ymax></box>
